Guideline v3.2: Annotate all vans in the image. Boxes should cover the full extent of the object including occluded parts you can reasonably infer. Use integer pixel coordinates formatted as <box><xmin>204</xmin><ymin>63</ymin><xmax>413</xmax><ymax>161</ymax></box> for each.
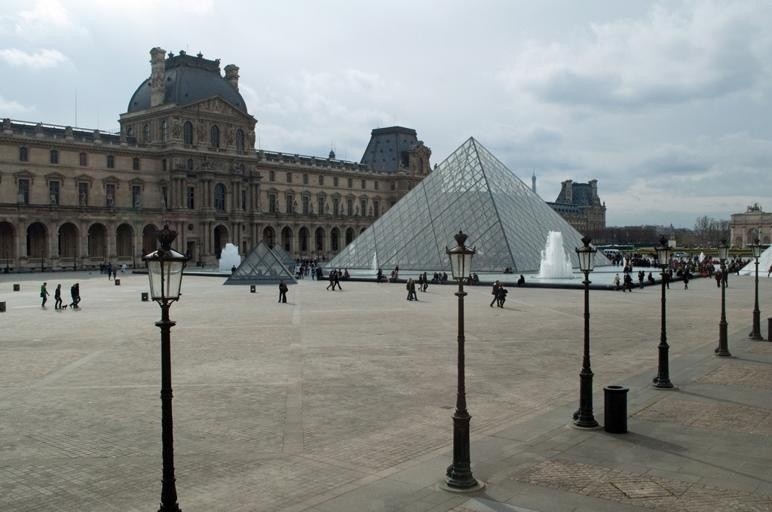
<box><xmin>603</xmin><ymin>249</ymin><xmax>619</xmax><ymax>257</ymax></box>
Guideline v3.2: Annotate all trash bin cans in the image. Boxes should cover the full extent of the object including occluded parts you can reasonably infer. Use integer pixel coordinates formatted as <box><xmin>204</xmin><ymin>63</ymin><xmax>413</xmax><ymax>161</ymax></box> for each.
<box><xmin>604</xmin><ymin>385</ymin><xmax>630</xmax><ymax>433</ymax></box>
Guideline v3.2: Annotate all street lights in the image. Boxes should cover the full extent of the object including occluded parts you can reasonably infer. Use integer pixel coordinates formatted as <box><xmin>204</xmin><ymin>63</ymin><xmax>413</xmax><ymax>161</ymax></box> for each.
<box><xmin>140</xmin><ymin>222</ymin><xmax>191</xmax><ymax>512</ymax></box>
<box><xmin>575</xmin><ymin>234</ymin><xmax>599</xmax><ymax>430</ymax></box>
<box><xmin>649</xmin><ymin>233</ymin><xmax>764</xmax><ymax>389</ymax></box>
<box><xmin>445</xmin><ymin>230</ymin><xmax>481</xmax><ymax>488</ymax></box>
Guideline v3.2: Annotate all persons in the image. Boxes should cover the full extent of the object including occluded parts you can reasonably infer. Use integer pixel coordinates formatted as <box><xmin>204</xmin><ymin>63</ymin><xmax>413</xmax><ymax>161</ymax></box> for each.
<box><xmin>97</xmin><ymin>262</ymin><xmax>129</xmax><ymax>282</ymax></box>
<box><xmin>70</xmin><ymin>282</ymin><xmax>77</xmax><ymax>310</ymax></box>
<box><xmin>371</xmin><ymin>264</ymin><xmax>528</xmax><ymax>309</ymax></box>
<box><xmin>326</xmin><ymin>267</ymin><xmax>351</xmax><ymax>291</ymax></box>
<box><xmin>603</xmin><ymin>248</ymin><xmax>752</xmax><ymax>294</ymax></box>
<box><xmin>52</xmin><ymin>282</ymin><xmax>64</xmax><ymax>310</ymax></box>
<box><xmin>292</xmin><ymin>256</ymin><xmax>325</xmax><ymax>281</ymax></box>
<box><xmin>74</xmin><ymin>282</ymin><xmax>81</xmax><ymax>309</ymax></box>
<box><xmin>282</xmin><ymin>282</ymin><xmax>288</xmax><ymax>303</ymax></box>
<box><xmin>40</xmin><ymin>280</ymin><xmax>49</xmax><ymax>309</ymax></box>
<box><xmin>277</xmin><ymin>280</ymin><xmax>285</xmax><ymax>303</ymax></box>
